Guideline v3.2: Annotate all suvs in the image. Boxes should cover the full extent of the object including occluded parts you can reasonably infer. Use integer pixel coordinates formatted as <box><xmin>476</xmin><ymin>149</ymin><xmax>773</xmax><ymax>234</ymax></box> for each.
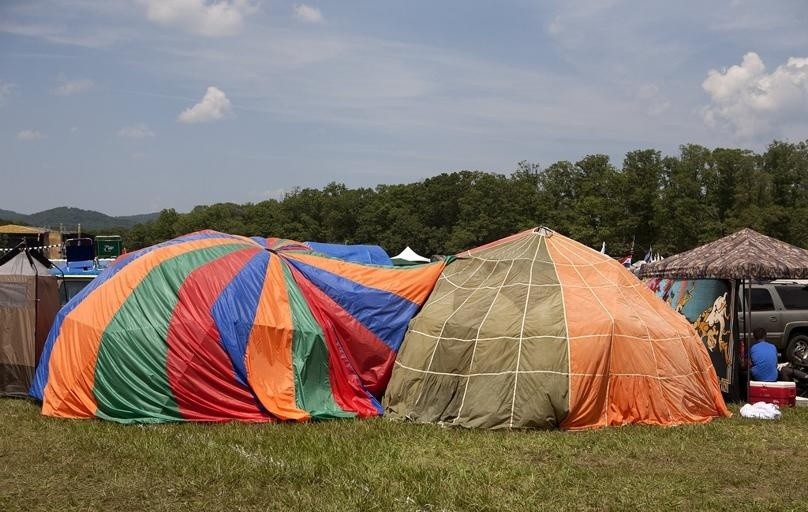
<box><xmin>737</xmin><ymin>277</ymin><xmax>808</xmax><ymax>370</ymax></box>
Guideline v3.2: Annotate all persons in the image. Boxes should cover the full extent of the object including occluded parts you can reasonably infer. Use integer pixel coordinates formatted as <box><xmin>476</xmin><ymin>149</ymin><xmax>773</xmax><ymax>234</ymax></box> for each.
<box><xmin>740</xmin><ymin>326</ymin><xmax>782</xmax><ymax>402</ymax></box>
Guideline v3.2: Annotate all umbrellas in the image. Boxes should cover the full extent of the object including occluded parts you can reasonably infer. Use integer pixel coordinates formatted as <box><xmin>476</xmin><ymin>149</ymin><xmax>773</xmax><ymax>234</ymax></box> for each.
<box><xmin>638</xmin><ymin>226</ymin><xmax>808</xmax><ymax>402</ymax></box>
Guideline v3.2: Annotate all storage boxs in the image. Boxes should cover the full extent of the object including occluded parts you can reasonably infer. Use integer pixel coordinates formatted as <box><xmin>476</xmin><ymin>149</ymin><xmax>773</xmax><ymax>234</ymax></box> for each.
<box><xmin>748</xmin><ymin>378</ymin><xmax>799</xmax><ymax>410</ymax></box>
